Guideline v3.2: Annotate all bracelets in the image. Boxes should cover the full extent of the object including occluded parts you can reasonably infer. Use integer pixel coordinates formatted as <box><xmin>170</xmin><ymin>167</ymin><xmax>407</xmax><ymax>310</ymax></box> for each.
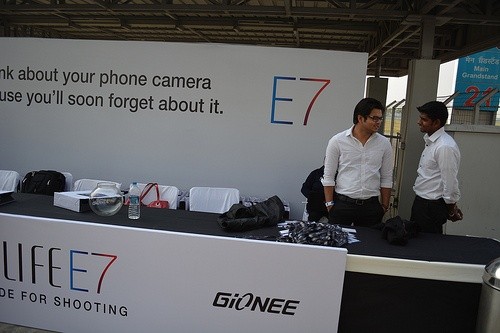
<box><xmin>324</xmin><ymin>201</ymin><xmax>335</xmax><ymax>207</ymax></box>
<box><xmin>383</xmin><ymin>204</ymin><xmax>388</xmax><ymax>212</ymax></box>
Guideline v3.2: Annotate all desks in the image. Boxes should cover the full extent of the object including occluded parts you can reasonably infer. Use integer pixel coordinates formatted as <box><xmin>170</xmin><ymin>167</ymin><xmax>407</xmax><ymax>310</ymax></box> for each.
<box><xmin>0</xmin><ymin>193</ymin><xmax>500</xmax><ymax>332</ymax></box>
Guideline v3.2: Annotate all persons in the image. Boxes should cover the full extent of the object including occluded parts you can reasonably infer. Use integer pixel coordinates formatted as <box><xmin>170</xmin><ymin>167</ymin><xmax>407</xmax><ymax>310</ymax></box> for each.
<box><xmin>409</xmin><ymin>101</ymin><xmax>463</xmax><ymax>235</ymax></box>
<box><xmin>320</xmin><ymin>97</ymin><xmax>394</xmax><ymax>226</ymax></box>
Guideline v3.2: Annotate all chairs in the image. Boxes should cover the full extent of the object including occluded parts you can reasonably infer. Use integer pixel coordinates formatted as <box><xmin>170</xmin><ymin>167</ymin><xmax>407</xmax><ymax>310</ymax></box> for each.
<box><xmin>129</xmin><ymin>183</ymin><xmax>180</xmax><ymax>209</ymax></box>
<box><xmin>0</xmin><ymin>170</ymin><xmax>19</xmax><ymax>192</ymax></box>
<box><xmin>74</xmin><ymin>179</ymin><xmax>121</xmax><ymax>193</ymax></box>
<box><xmin>189</xmin><ymin>187</ymin><xmax>239</xmax><ymax>213</ymax></box>
<box><xmin>62</xmin><ymin>172</ymin><xmax>73</xmax><ymax>191</ymax></box>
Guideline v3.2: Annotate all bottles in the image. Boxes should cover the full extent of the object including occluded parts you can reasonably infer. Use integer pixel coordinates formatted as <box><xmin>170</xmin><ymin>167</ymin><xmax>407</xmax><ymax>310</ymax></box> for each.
<box><xmin>128</xmin><ymin>182</ymin><xmax>140</xmax><ymax>219</ymax></box>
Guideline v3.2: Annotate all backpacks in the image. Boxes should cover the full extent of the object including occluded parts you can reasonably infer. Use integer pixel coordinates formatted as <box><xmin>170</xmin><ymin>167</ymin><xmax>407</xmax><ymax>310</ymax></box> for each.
<box><xmin>20</xmin><ymin>170</ymin><xmax>66</xmax><ymax>194</ymax></box>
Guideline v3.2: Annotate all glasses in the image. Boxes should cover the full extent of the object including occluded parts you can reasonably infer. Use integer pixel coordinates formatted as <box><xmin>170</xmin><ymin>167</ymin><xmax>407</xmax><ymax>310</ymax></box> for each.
<box><xmin>368</xmin><ymin>115</ymin><xmax>385</xmax><ymax>120</ymax></box>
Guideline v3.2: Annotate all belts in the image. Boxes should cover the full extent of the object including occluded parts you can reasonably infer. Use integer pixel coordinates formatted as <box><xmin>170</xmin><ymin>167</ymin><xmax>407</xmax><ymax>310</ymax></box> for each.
<box><xmin>337</xmin><ymin>195</ymin><xmax>378</xmax><ymax>206</ymax></box>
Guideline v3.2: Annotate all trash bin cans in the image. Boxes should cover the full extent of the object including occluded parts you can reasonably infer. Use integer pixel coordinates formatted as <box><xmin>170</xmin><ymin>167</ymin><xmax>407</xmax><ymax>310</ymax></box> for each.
<box><xmin>481</xmin><ymin>259</ymin><xmax>500</xmax><ymax>332</ymax></box>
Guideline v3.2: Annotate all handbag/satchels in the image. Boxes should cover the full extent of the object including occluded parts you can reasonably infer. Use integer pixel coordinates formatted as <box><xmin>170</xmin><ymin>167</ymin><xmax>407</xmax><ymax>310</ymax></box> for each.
<box><xmin>124</xmin><ymin>183</ymin><xmax>169</xmax><ymax>209</ymax></box>
